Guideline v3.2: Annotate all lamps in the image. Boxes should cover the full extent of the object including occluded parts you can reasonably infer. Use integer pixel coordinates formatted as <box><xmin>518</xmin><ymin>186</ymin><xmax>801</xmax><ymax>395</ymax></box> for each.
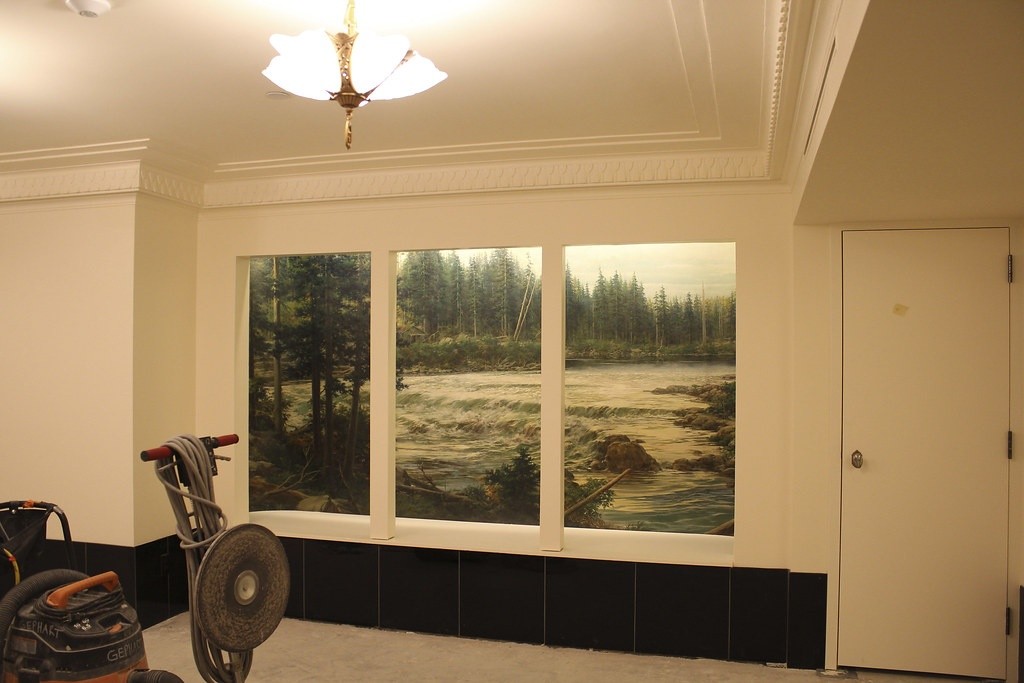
<box><xmin>263</xmin><ymin>0</ymin><xmax>448</xmax><ymax>149</ymax></box>
